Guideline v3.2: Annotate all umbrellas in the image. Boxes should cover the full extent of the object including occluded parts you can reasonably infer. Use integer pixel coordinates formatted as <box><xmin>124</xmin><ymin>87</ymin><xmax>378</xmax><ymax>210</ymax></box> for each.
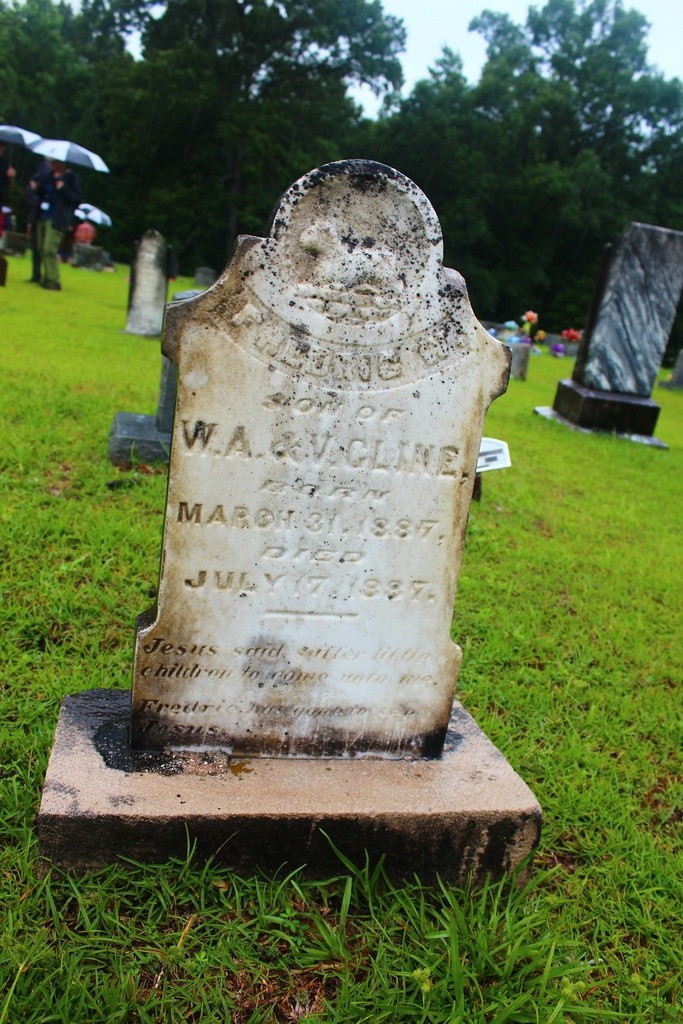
<box><xmin>0</xmin><ymin>125</ymin><xmax>111</xmax><ymax>173</ymax></box>
<box><xmin>74</xmin><ymin>203</ymin><xmax>111</xmax><ymax>226</ymax></box>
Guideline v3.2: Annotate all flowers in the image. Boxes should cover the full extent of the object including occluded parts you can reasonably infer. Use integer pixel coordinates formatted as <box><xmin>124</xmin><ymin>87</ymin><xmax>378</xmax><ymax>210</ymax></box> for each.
<box><xmin>486</xmin><ymin>310</ymin><xmax>581</xmax><ymax>358</ymax></box>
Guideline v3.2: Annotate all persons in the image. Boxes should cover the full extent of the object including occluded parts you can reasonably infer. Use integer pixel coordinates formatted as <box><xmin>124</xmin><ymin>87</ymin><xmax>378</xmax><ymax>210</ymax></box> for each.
<box><xmin>0</xmin><ymin>142</ymin><xmax>16</xmax><ymax>239</ymax></box>
<box><xmin>26</xmin><ymin>160</ymin><xmax>80</xmax><ymax>291</ymax></box>
<box><xmin>59</xmin><ymin>221</ymin><xmax>96</xmax><ymax>261</ymax></box>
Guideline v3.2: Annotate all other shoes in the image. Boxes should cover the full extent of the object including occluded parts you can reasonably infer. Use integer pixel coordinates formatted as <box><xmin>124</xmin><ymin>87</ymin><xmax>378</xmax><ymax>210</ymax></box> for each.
<box><xmin>29</xmin><ymin>277</ymin><xmax>40</xmax><ymax>282</ymax></box>
